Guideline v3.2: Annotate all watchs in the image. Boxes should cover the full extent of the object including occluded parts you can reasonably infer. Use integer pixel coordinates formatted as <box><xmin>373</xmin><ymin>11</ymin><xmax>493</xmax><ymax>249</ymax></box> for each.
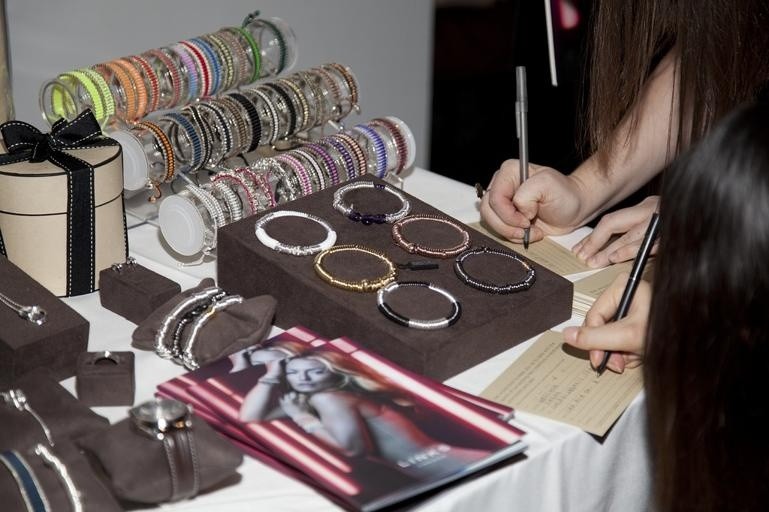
<box><xmin>128</xmin><ymin>396</ymin><xmax>201</xmax><ymax>505</ymax></box>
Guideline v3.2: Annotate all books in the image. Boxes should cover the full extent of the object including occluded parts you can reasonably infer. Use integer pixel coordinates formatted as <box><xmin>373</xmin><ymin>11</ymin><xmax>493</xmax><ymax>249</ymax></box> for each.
<box><xmin>573</xmin><ymin>255</ymin><xmax>655</xmax><ymax>313</ymax></box>
<box><xmin>152</xmin><ymin>337</ymin><xmax>527</xmax><ymax>511</ymax></box>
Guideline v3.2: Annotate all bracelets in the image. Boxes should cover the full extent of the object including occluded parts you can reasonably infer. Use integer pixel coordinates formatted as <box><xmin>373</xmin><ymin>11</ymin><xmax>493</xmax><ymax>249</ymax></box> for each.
<box><xmin>376</xmin><ymin>281</ymin><xmax>463</xmax><ymax>327</ymax></box>
<box><xmin>312</xmin><ymin>245</ymin><xmax>397</xmax><ymax>292</ymax></box>
<box><xmin>391</xmin><ymin>215</ymin><xmax>472</xmax><ymax>258</ymax></box>
<box><xmin>1</xmin><ymin>387</ymin><xmax>87</xmax><ymax>512</ymax></box>
<box><xmin>153</xmin><ymin>284</ymin><xmax>243</xmax><ymax>371</ymax></box>
<box><xmin>38</xmin><ymin>15</ymin><xmax>418</xmax><ymax>255</ymax></box>
<box><xmin>453</xmin><ymin>247</ymin><xmax>536</xmax><ymax>294</ymax></box>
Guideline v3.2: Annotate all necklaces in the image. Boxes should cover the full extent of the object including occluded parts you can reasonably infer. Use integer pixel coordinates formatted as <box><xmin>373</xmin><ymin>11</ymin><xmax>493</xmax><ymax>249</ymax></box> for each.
<box><xmin>0</xmin><ymin>292</ymin><xmax>48</xmax><ymax>330</ymax></box>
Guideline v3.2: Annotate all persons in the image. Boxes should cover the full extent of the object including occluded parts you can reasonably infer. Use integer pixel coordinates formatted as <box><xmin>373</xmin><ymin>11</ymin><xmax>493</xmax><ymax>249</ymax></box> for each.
<box><xmin>242</xmin><ymin>351</ymin><xmax>490</xmax><ymax>482</ymax></box>
<box><xmin>563</xmin><ymin>116</ymin><xmax>769</xmax><ymax>511</ymax></box>
<box><xmin>480</xmin><ymin>0</ymin><xmax>769</xmax><ymax>270</ymax></box>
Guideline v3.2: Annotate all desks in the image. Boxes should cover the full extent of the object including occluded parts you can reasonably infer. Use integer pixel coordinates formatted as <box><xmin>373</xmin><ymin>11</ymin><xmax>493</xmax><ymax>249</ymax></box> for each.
<box><xmin>54</xmin><ymin>129</ymin><xmax>657</xmax><ymax>512</ymax></box>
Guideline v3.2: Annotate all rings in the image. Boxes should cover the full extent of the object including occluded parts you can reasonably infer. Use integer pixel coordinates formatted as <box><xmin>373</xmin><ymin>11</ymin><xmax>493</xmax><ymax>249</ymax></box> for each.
<box><xmin>126</xmin><ymin>256</ymin><xmax>137</xmax><ymax>272</ymax></box>
<box><xmin>93</xmin><ymin>348</ymin><xmax>120</xmax><ymax>364</ymax></box>
<box><xmin>475</xmin><ymin>183</ymin><xmax>491</xmax><ymax>199</ymax></box>
<box><xmin>112</xmin><ymin>263</ymin><xmax>125</xmax><ymax>274</ymax></box>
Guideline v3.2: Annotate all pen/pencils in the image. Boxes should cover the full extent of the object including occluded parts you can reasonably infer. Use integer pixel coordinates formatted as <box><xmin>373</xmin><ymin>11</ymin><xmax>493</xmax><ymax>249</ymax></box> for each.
<box><xmin>597</xmin><ymin>213</ymin><xmax>660</xmax><ymax>378</ymax></box>
<box><xmin>516</xmin><ymin>66</ymin><xmax>529</xmax><ymax>252</ymax></box>
